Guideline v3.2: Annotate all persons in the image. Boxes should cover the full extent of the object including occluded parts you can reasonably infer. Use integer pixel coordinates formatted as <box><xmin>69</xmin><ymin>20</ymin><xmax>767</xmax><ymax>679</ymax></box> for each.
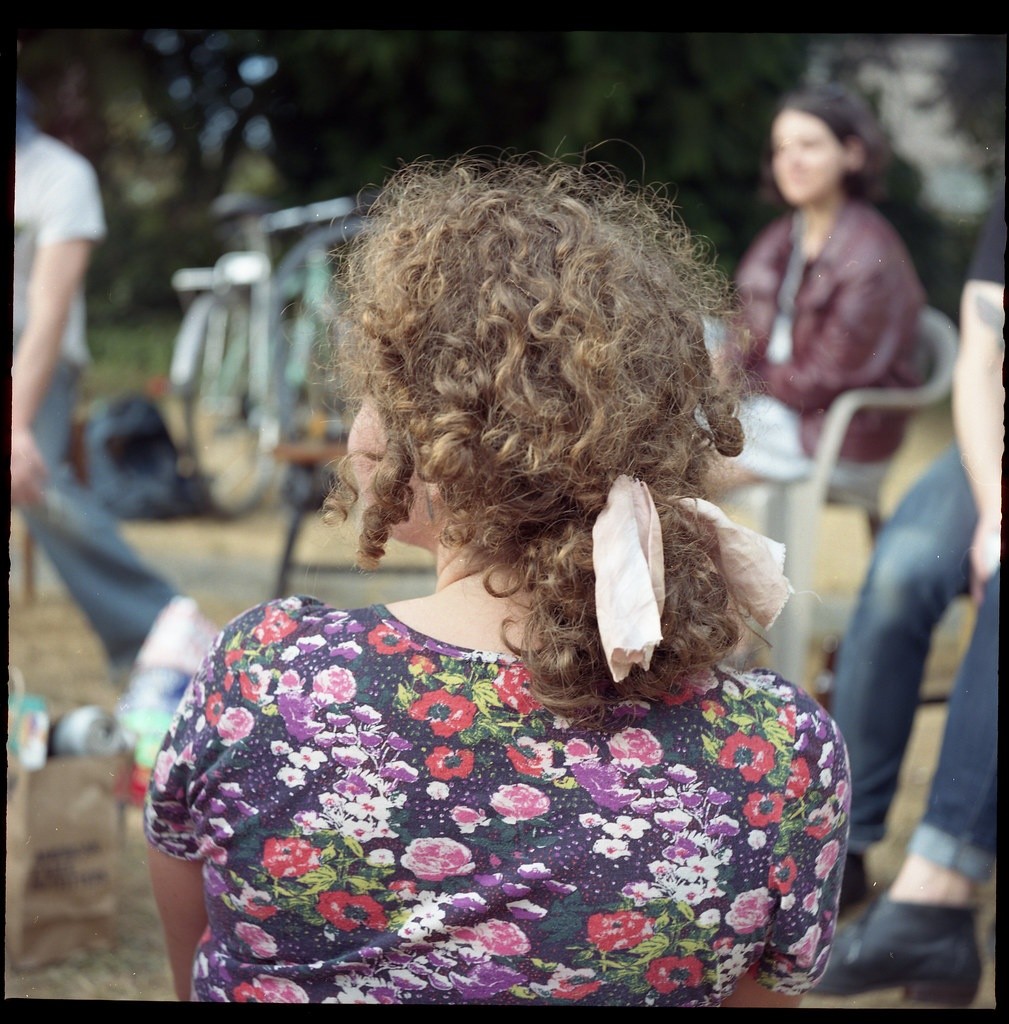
<box><xmin>142</xmin><ymin>164</ymin><xmax>852</xmax><ymax>1007</ymax></box>
<box><xmin>808</xmin><ymin>198</ymin><xmax>1005</xmax><ymax>1005</ymax></box>
<box><xmin>12</xmin><ymin>27</ymin><xmax>202</xmax><ymax>674</ymax></box>
<box><xmin>709</xmin><ymin>86</ymin><xmax>922</xmax><ymax>495</ymax></box>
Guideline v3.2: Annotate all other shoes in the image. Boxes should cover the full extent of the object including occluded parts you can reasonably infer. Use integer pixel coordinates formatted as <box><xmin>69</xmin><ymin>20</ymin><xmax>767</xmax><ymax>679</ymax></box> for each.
<box><xmin>804</xmin><ymin>894</ymin><xmax>982</xmax><ymax>1007</ymax></box>
<box><xmin>841</xmin><ymin>855</ymin><xmax>867</xmax><ymax>912</ymax></box>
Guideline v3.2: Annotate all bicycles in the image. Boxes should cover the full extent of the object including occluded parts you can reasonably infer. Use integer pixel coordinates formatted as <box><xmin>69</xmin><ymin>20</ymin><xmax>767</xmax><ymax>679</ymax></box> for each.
<box><xmin>171</xmin><ymin>188</ymin><xmax>283</xmax><ymax>524</ymax></box>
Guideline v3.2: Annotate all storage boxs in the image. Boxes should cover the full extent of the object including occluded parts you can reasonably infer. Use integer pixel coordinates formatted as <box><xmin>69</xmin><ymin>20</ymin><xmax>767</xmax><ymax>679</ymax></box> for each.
<box><xmin>4</xmin><ymin>748</ymin><xmax>138</xmax><ymax>978</ymax></box>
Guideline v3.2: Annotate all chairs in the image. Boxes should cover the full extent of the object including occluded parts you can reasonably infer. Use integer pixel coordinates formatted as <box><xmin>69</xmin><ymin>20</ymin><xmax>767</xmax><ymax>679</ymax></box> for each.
<box><xmin>758</xmin><ymin>303</ymin><xmax>955</xmax><ymax>690</ymax></box>
